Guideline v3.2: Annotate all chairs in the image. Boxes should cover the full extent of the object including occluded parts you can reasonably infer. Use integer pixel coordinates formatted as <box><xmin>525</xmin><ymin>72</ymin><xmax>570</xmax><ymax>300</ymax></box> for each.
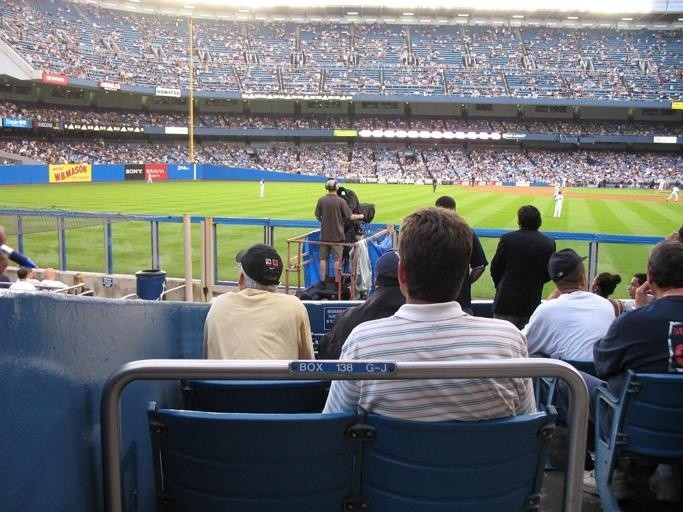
<box><xmin>0</xmin><ymin>0</ymin><xmax>683</xmax><ymax>189</ymax></box>
<box><xmin>0</xmin><ymin>252</ymin><xmax>683</xmax><ymax>511</ymax></box>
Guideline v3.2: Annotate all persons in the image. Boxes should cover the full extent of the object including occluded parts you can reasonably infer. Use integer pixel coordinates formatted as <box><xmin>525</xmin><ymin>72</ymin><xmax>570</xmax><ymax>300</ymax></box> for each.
<box><xmin>315</xmin><ymin>180</ymin><xmax>363</xmax><ymax>291</ymax></box>
<box><xmin>204</xmin><ymin>243</ymin><xmax>316</xmax><ymax>359</ymax></box>
<box><xmin>0</xmin><ymin>0</ymin><xmax>682</xmax><ymax>191</ymax></box>
<box><xmin>0</xmin><ymin>228</ymin><xmax>93</xmax><ymax>296</ymax></box>
<box><xmin>317</xmin><ymin>197</ymin><xmax>557</xmax><ymax>422</ymax></box>
<box><xmin>520</xmin><ymin>228</ymin><xmax>683</xmax><ymax>500</ymax></box>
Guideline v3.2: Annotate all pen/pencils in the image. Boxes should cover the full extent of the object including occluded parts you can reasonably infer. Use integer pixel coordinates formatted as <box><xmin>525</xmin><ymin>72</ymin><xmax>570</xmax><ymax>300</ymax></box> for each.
<box><xmin>0</xmin><ymin>244</ymin><xmax>40</xmax><ymax>270</ymax></box>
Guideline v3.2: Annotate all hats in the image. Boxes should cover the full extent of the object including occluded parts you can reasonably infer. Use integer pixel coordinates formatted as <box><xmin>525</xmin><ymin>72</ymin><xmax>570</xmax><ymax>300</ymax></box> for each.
<box><xmin>547</xmin><ymin>248</ymin><xmax>587</xmax><ymax>281</ymax></box>
<box><xmin>375</xmin><ymin>250</ymin><xmax>400</xmax><ymax>286</ymax></box>
<box><xmin>235</xmin><ymin>244</ymin><xmax>284</xmax><ymax>286</ymax></box>
<box><xmin>326</xmin><ymin>179</ymin><xmax>337</xmax><ymax>190</ymax></box>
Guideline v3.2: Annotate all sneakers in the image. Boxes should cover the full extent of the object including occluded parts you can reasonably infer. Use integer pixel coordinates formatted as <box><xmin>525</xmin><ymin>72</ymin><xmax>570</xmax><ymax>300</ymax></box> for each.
<box><xmin>650</xmin><ymin>467</ymin><xmax>678</xmax><ymax>502</ymax></box>
<box><xmin>581</xmin><ymin>468</ymin><xmax>632</xmax><ymax>500</ymax></box>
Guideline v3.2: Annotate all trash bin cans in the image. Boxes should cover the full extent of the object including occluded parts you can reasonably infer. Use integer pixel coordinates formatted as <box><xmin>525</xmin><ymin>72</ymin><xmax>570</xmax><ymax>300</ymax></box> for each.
<box><xmin>135</xmin><ymin>270</ymin><xmax>167</xmax><ymax>302</ymax></box>
